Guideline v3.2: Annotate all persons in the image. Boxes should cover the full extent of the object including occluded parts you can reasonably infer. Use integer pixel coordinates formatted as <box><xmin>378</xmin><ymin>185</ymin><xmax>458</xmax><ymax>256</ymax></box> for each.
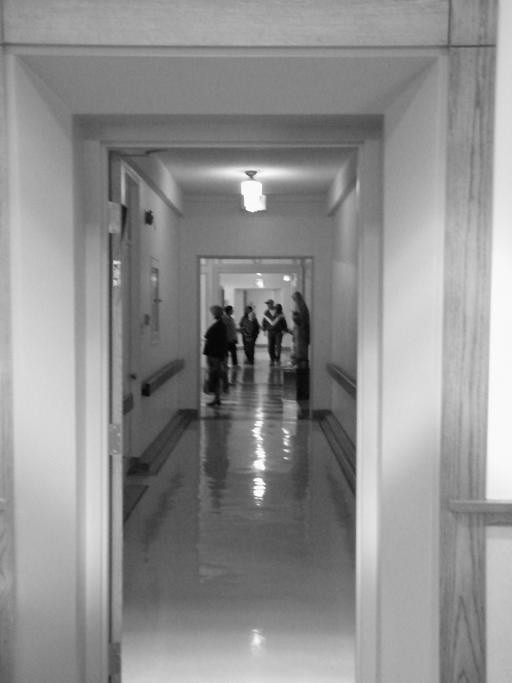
<box><xmin>201</xmin><ymin>291</ymin><xmax>309</xmax><ymax>406</ymax></box>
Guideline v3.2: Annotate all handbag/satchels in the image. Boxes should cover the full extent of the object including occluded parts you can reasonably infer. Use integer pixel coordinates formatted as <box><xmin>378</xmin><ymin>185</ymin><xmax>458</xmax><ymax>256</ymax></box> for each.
<box><xmin>203</xmin><ymin>367</ymin><xmax>224</xmax><ymax>395</ymax></box>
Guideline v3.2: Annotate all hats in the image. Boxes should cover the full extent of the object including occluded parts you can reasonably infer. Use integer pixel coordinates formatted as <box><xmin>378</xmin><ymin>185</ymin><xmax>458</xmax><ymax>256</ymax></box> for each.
<box><xmin>264</xmin><ymin>299</ymin><xmax>273</xmax><ymax>304</ymax></box>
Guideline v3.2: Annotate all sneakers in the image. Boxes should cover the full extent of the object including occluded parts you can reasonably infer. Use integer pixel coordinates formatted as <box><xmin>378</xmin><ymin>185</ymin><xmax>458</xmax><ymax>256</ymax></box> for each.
<box><xmin>207</xmin><ymin>399</ymin><xmax>222</xmax><ymax>406</ymax></box>
